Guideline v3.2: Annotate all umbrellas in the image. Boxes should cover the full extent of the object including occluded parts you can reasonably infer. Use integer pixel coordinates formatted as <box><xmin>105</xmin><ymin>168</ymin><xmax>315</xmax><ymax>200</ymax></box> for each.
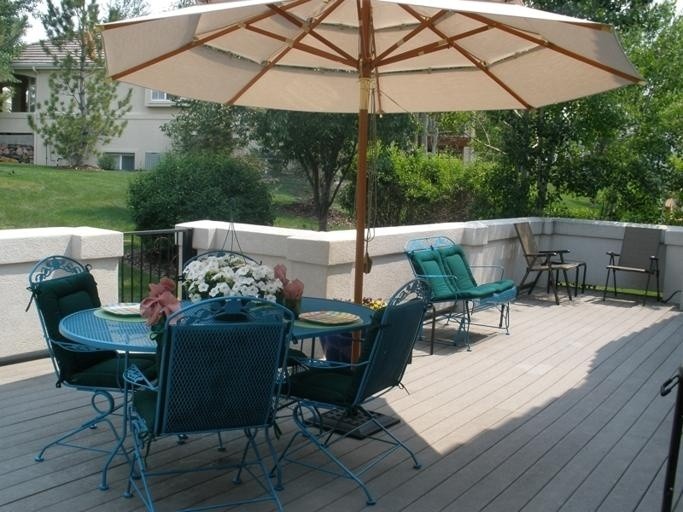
<box><xmin>97</xmin><ymin>0</ymin><xmax>648</xmax><ymax>415</ymax></box>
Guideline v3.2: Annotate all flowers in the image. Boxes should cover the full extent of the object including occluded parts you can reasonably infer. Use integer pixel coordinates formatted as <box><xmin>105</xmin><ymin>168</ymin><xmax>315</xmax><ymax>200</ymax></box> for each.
<box><xmin>334</xmin><ymin>297</ymin><xmax>387</xmax><ymax>315</ymax></box>
<box><xmin>184</xmin><ymin>254</ymin><xmax>283</xmax><ymax>311</ymax></box>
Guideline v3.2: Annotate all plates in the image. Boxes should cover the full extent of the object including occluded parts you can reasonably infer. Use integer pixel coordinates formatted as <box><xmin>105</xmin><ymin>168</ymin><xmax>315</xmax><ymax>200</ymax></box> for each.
<box><xmin>101</xmin><ymin>302</ymin><xmax>142</xmax><ymax>315</ymax></box>
<box><xmin>298</xmin><ymin>310</ymin><xmax>360</xmax><ymax>325</ymax></box>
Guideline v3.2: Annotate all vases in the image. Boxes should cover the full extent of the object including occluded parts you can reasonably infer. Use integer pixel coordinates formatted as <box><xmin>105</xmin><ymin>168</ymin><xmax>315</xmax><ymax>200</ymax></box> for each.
<box><xmin>317</xmin><ymin>328</ymin><xmax>386</xmax><ymax>364</ymax></box>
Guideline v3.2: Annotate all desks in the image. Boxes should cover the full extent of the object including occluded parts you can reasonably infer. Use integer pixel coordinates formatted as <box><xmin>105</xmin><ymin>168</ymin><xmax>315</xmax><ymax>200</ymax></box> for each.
<box><xmin>57</xmin><ymin>295</ymin><xmax>377</xmax><ymax>442</ymax></box>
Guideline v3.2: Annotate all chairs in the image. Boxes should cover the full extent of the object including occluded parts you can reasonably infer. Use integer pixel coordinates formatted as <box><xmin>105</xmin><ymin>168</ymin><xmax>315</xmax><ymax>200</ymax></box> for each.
<box><xmin>25</xmin><ymin>254</ymin><xmax>160</xmax><ymax>489</ymax></box>
<box><xmin>277</xmin><ymin>276</ymin><xmax>435</xmax><ymax>506</ymax></box>
<box><xmin>602</xmin><ymin>225</ymin><xmax>661</xmax><ymax>306</ymax></box>
<box><xmin>515</xmin><ymin>222</ymin><xmax>586</xmax><ymax>304</ymax></box>
<box><xmin>182</xmin><ymin>250</ymin><xmax>264</xmax><ymax>268</ymax></box>
<box><xmin>118</xmin><ymin>295</ymin><xmax>293</xmax><ymax>512</ymax></box>
<box><xmin>404</xmin><ymin>236</ymin><xmax>517</xmax><ymax>352</ymax></box>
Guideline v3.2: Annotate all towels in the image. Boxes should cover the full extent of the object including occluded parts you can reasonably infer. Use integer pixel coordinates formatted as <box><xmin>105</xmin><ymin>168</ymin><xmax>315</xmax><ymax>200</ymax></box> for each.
<box><xmin>139</xmin><ymin>282</ymin><xmax>183</xmax><ymax>327</ymax></box>
<box><xmin>273</xmin><ymin>263</ymin><xmax>287</xmax><ymax>287</ymax></box>
<box><xmin>285</xmin><ymin>279</ymin><xmax>303</xmax><ymax>302</ymax></box>
<box><xmin>159</xmin><ymin>276</ymin><xmax>177</xmax><ymax>295</ymax></box>
